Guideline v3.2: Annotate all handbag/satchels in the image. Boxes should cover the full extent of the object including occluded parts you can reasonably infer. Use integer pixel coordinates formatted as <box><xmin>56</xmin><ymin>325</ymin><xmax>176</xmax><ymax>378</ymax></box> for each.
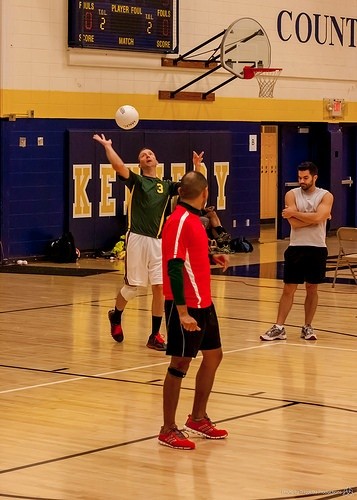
<box><xmin>217</xmin><ymin>233</ymin><xmax>231</xmax><ymax>244</ymax></box>
<box><xmin>230</xmin><ymin>236</ymin><xmax>253</xmax><ymax>252</ymax></box>
<box><xmin>51</xmin><ymin>231</ymin><xmax>77</xmax><ymax>263</ymax></box>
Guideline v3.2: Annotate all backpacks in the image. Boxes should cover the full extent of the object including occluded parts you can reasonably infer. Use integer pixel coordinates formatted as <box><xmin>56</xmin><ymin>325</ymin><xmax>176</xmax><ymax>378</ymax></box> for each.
<box><xmin>111</xmin><ymin>235</ymin><xmax>126</xmax><ymax>257</ymax></box>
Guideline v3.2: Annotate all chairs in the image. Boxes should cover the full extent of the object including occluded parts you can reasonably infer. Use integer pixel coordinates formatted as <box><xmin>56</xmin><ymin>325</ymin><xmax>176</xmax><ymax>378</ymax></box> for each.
<box><xmin>332</xmin><ymin>226</ymin><xmax>357</xmax><ymax>289</ymax></box>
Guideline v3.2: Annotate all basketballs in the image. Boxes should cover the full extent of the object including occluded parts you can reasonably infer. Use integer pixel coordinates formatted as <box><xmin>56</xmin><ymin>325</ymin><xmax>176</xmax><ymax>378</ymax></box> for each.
<box><xmin>115</xmin><ymin>105</ymin><xmax>139</xmax><ymax>129</ymax></box>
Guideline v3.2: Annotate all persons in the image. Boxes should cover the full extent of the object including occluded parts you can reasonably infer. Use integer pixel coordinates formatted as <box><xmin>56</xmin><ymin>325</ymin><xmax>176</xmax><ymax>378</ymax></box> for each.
<box><xmin>92</xmin><ymin>133</ymin><xmax>204</xmax><ymax>351</ymax></box>
<box><xmin>259</xmin><ymin>162</ymin><xmax>333</xmax><ymax>341</ymax></box>
<box><xmin>157</xmin><ymin>171</ymin><xmax>231</xmax><ymax>451</ymax></box>
<box><xmin>177</xmin><ymin>196</ymin><xmax>230</xmax><ymax>243</ymax></box>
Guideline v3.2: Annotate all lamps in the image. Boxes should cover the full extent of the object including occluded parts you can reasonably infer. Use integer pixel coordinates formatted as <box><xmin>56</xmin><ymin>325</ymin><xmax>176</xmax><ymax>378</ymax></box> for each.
<box><xmin>327</xmin><ymin>98</ymin><xmax>346</xmax><ymax>118</ymax></box>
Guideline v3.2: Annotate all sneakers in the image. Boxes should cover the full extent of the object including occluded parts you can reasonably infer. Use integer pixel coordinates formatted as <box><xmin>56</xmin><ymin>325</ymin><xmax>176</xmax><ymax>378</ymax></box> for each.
<box><xmin>146</xmin><ymin>334</ymin><xmax>168</xmax><ymax>350</ymax></box>
<box><xmin>260</xmin><ymin>325</ymin><xmax>288</xmax><ymax>340</ymax></box>
<box><xmin>300</xmin><ymin>325</ymin><xmax>317</xmax><ymax>340</ymax></box>
<box><xmin>108</xmin><ymin>310</ymin><xmax>125</xmax><ymax>343</ymax></box>
<box><xmin>158</xmin><ymin>425</ymin><xmax>196</xmax><ymax>450</ymax></box>
<box><xmin>184</xmin><ymin>413</ymin><xmax>228</xmax><ymax>439</ymax></box>
